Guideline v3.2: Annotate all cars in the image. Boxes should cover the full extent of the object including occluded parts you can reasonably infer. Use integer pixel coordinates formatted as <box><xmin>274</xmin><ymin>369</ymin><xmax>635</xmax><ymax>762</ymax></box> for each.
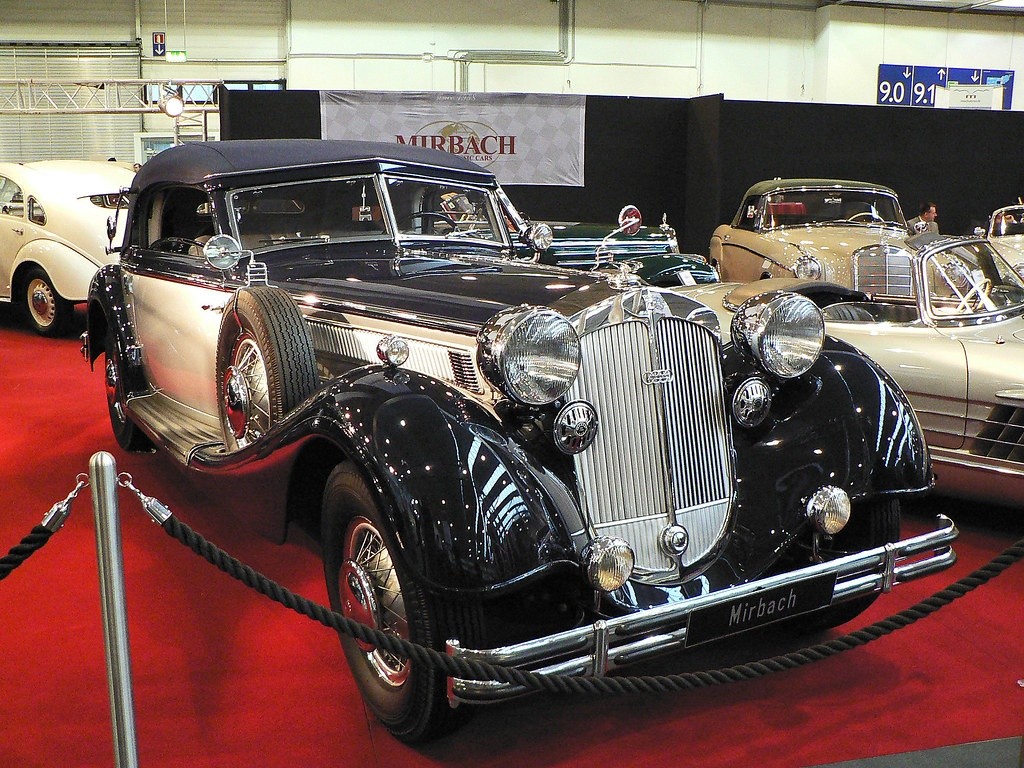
<box><xmin>0</xmin><ymin>160</ymin><xmax>138</xmax><ymax>335</ymax></box>
<box><xmin>436</xmin><ymin>193</ymin><xmax>719</xmax><ymax>288</ymax></box>
<box><xmin>709</xmin><ymin>179</ymin><xmax>971</xmax><ymax>299</ymax></box>
<box><xmin>79</xmin><ymin>139</ymin><xmax>959</xmax><ymax>745</ymax></box>
<box><xmin>973</xmin><ymin>204</ymin><xmax>1023</xmax><ymax>284</ymax></box>
<box><xmin>668</xmin><ymin>236</ymin><xmax>1024</xmax><ymax>508</ymax></box>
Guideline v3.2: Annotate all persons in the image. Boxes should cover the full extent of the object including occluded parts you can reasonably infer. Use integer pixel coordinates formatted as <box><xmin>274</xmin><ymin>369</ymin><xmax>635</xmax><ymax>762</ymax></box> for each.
<box><xmin>906</xmin><ymin>202</ymin><xmax>939</xmax><ymax>236</ymax></box>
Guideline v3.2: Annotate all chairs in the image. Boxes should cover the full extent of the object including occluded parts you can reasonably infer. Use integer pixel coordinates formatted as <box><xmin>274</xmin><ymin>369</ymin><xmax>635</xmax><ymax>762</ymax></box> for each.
<box><xmin>767</xmin><ymin>202</ymin><xmax>805</xmax><ymax>227</ymax></box>
<box><xmin>189</xmin><ymin>229</ymin><xmax>273</xmax><ymax>257</ymax></box>
<box><xmin>822</xmin><ymin>303</ymin><xmax>875</xmax><ymax>322</ymax></box>
<box><xmin>836</xmin><ymin>202</ymin><xmax>875</xmax><ymax>222</ymax></box>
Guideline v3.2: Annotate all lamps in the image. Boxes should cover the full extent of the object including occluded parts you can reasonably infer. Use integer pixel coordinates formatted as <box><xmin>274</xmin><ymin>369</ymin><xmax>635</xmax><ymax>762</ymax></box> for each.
<box><xmin>160</xmin><ymin>85</ymin><xmax>184</xmax><ymax>117</ymax></box>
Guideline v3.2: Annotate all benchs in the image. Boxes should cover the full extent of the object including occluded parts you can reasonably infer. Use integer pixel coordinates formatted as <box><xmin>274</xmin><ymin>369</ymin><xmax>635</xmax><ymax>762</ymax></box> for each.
<box><xmin>267</xmin><ymin>221</ymin><xmax>382</xmax><ymax>244</ymax></box>
